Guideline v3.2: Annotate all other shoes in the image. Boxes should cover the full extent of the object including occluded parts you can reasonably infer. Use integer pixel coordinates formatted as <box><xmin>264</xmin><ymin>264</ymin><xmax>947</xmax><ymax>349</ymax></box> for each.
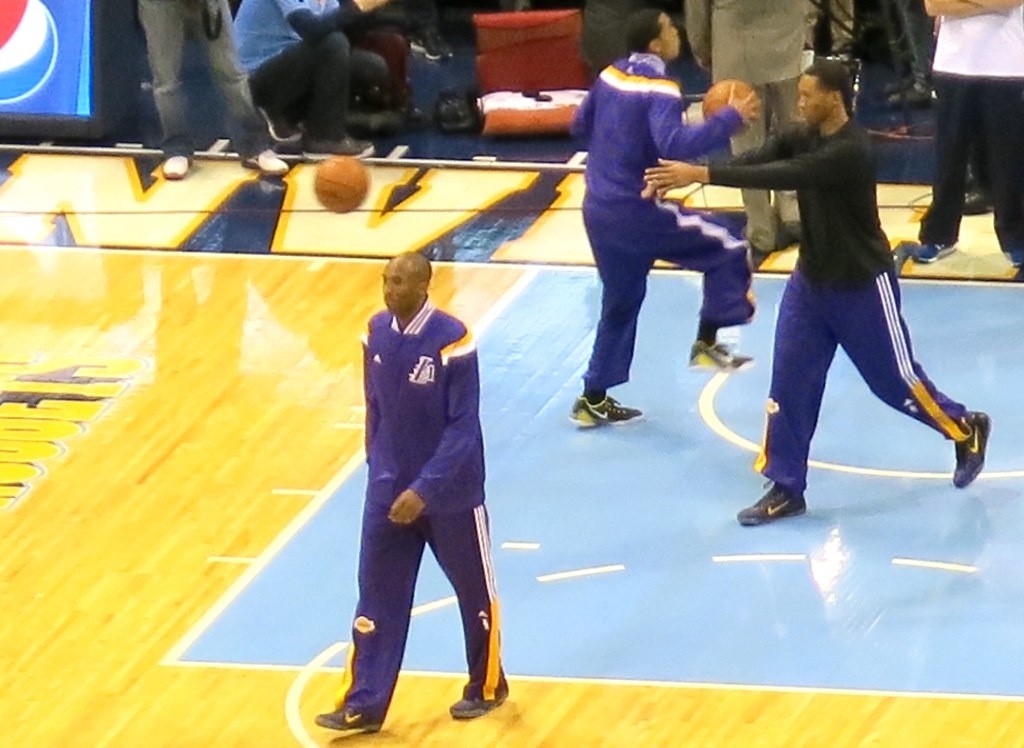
<box><xmin>741</xmin><ymin>216</ymin><xmax>802</xmax><ymax>257</ymax></box>
<box><xmin>163</xmin><ymin>155</ymin><xmax>189</xmax><ymax>179</ymax></box>
<box><xmin>242</xmin><ymin>149</ymin><xmax>289</xmax><ymax>177</ymax></box>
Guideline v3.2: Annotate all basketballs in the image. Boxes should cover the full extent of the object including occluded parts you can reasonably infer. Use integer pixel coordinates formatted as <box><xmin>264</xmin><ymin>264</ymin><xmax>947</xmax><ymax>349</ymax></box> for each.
<box><xmin>312</xmin><ymin>154</ymin><xmax>373</xmax><ymax>213</ymax></box>
<box><xmin>700</xmin><ymin>79</ymin><xmax>759</xmax><ymax>136</ymax></box>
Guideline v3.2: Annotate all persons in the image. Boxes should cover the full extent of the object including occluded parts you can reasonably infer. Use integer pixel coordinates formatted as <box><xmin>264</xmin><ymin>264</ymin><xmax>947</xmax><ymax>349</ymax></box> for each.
<box><xmin>137</xmin><ymin>0</ymin><xmax>454</xmax><ymax>180</ymax></box>
<box><xmin>642</xmin><ymin>62</ymin><xmax>990</xmax><ymax>527</ymax></box>
<box><xmin>568</xmin><ymin>9</ymin><xmax>763</xmax><ymax>428</ymax></box>
<box><xmin>912</xmin><ymin>0</ymin><xmax>1024</xmax><ymax>268</ymax></box>
<box><xmin>683</xmin><ymin>0</ymin><xmax>817</xmax><ymax>267</ymax></box>
<box><xmin>313</xmin><ymin>253</ymin><xmax>507</xmax><ymax>734</ymax></box>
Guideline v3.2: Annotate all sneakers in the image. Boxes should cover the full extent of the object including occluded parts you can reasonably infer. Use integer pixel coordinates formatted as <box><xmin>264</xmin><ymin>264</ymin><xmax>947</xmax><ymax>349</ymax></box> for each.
<box><xmin>737</xmin><ymin>479</ymin><xmax>806</xmax><ymax>524</ymax></box>
<box><xmin>303</xmin><ymin>135</ymin><xmax>375</xmax><ymax>161</ymax></box>
<box><xmin>953</xmin><ymin>412</ymin><xmax>989</xmax><ymax>488</ymax></box>
<box><xmin>315</xmin><ymin>705</ymin><xmax>384</xmax><ymax>732</ymax></box>
<box><xmin>569</xmin><ymin>395</ymin><xmax>643</xmax><ymax>427</ymax></box>
<box><xmin>450</xmin><ymin>689</ymin><xmax>508</xmax><ymax>718</ymax></box>
<box><xmin>259</xmin><ymin>106</ymin><xmax>302</xmax><ymax>143</ymax></box>
<box><xmin>912</xmin><ymin>242</ymin><xmax>957</xmax><ymax>263</ymax></box>
<box><xmin>688</xmin><ymin>340</ymin><xmax>755</xmax><ymax>372</ymax></box>
<box><xmin>1006</xmin><ymin>250</ymin><xmax>1024</xmax><ymax>267</ymax></box>
